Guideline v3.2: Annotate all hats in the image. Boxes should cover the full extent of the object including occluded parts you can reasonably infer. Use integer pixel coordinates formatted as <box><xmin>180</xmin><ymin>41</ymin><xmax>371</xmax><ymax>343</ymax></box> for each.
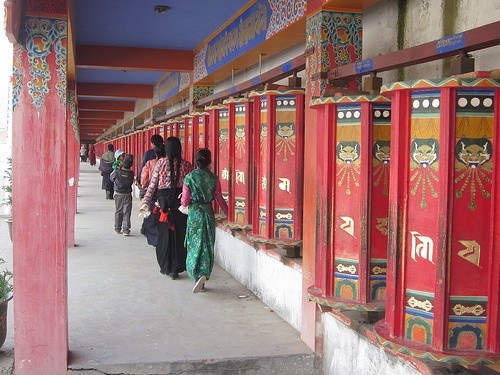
<box><xmin>114</xmin><ymin>149</ymin><xmax>125</xmax><ymax>160</ymax></box>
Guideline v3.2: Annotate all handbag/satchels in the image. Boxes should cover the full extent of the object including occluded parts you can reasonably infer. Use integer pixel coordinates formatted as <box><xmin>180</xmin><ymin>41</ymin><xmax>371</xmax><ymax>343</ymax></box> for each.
<box><xmin>140</xmin><ymin>218</ymin><xmax>148</xmax><ymax>235</ymax></box>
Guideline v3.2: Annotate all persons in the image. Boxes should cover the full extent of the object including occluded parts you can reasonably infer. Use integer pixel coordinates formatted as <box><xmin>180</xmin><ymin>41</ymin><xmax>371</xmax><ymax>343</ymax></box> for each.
<box><xmin>140</xmin><ymin>136</ymin><xmax>195</xmax><ymax>280</ymax></box>
<box><xmin>98</xmin><ymin>144</ymin><xmax>116</xmax><ymax>199</ymax></box>
<box><xmin>110</xmin><ymin>134</ymin><xmax>166</xmax><ymax>247</ymax></box>
<box><xmin>180</xmin><ymin>148</ymin><xmax>229</xmax><ymax>293</ymax></box>
<box><xmin>80</xmin><ymin>144</ymin><xmax>96</xmax><ymax>167</ymax></box>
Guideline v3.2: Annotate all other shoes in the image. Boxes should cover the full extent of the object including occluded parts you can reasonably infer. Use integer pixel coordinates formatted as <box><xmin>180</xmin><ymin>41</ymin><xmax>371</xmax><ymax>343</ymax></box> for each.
<box><xmin>168</xmin><ymin>272</ymin><xmax>178</xmax><ymax>281</ymax></box>
<box><xmin>123</xmin><ymin>230</ymin><xmax>130</xmax><ymax>236</ymax></box>
<box><xmin>192</xmin><ymin>276</ymin><xmax>206</xmax><ymax>294</ymax></box>
